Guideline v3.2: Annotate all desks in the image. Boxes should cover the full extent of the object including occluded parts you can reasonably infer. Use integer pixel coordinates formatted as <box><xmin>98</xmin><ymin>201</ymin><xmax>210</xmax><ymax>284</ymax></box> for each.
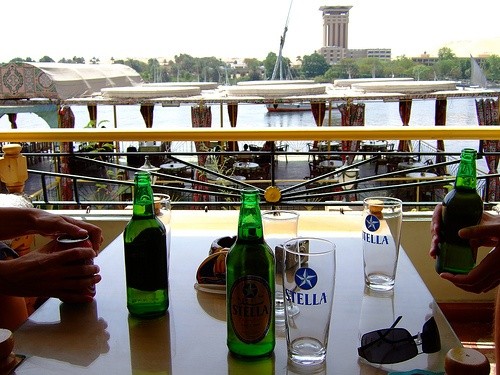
<box><xmin>157</xmin><ymin>142</ymin><xmax>438</xmax><ymax>201</ymax></box>
<box><xmin>9</xmin><ymin>210</ymin><xmax>462</xmax><ymax>375</ymax></box>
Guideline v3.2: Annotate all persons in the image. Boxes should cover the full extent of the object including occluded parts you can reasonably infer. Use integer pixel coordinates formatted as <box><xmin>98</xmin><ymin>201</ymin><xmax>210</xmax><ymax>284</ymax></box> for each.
<box><xmin>0</xmin><ymin>207</ymin><xmax>104</xmax><ymax>333</ymax></box>
<box><xmin>216</xmin><ymin>140</ymin><xmax>272</xmax><ymax>163</ymax></box>
<box><xmin>430</xmin><ymin>203</ymin><xmax>500</xmax><ymax>294</ymax></box>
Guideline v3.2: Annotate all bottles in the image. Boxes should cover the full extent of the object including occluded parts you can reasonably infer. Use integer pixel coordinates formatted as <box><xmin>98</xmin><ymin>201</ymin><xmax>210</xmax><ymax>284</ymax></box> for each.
<box><xmin>123</xmin><ymin>171</ymin><xmax>170</xmax><ymax>317</ymax></box>
<box><xmin>435</xmin><ymin>148</ymin><xmax>483</xmax><ymax>277</ymax></box>
<box><xmin>225</xmin><ymin>189</ymin><xmax>276</xmax><ymax>359</ymax></box>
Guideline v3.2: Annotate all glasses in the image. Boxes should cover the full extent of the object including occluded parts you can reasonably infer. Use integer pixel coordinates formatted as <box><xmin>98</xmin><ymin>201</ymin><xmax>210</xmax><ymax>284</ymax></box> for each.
<box><xmin>358</xmin><ymin>315</ymin><xmax>441</xmax><ymax>364</ymax></box>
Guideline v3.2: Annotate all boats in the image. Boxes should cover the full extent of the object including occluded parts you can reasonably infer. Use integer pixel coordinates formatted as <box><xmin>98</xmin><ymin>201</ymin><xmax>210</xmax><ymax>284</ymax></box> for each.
<box><xmin>265</xmin><ymin>0</ymin><xmax>357</xmax><ymax>112</ymax></box>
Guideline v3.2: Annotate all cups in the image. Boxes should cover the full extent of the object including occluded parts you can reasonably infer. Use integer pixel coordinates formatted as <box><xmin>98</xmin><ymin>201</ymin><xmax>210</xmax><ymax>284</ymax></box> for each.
<box><xmin>152</xmin><ymin>192</ymin><xmax>171</xmax><ymax>274</ymax></box>
<box><xmin>286</xmin><ymin>363</ymin><xmax>327</xmax><ymax>375</ymax></box>
<box><xmin>262</xmin><ymin>210</ymin><xmax>299</xmax><ymax>317</ymax></box>
<box><xmin>281</xmin><ymin>236</ymin><xmax>337</xmax><ymax>366</ymax></box>
<box><xmin>361</xmin><ymin>196</ymin><xmax>403</xmax><ymax>291</ymax></box>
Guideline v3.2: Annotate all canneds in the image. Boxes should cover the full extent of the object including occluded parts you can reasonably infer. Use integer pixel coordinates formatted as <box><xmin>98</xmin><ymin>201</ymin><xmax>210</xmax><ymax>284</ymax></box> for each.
<box><xmin>59</xmin><ymin>300</ymin><xmax>98</xmax><ymax>323</ymax></box>
<box><xmin>56</xmin><ymin>233</ymin><xmax>96</xmax><ymax>304</ymax></box>
